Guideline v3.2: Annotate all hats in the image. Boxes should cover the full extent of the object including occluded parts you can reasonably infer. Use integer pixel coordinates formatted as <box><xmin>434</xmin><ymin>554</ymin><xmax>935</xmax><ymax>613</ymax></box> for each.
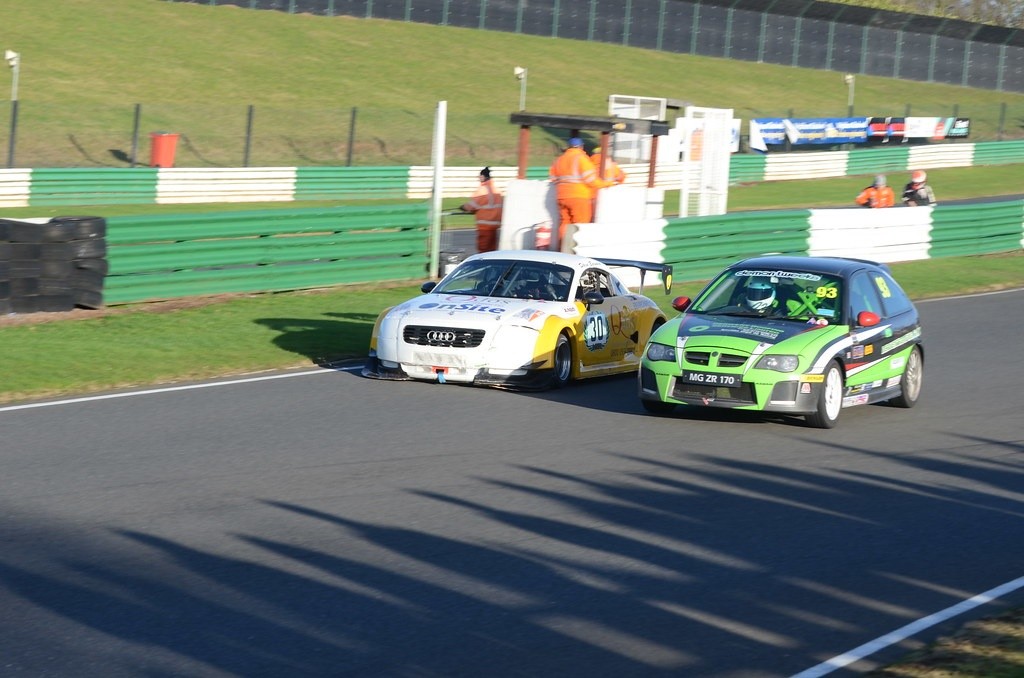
<box><xmin>567</xmin><ymin>137</ymin><xmax>585</xmax><ymax>148</ymax></box>
<box><xmin>480</xmin><ymin>166</ymin><xmax>490</xmax><ymax>177</ymax></box>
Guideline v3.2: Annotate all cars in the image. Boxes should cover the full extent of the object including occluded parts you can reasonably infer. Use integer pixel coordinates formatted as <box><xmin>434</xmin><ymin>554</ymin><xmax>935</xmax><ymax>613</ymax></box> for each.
<box><xmin>637</xmin><ymin>250</ymin><xmax>924</xmax><ymax>428</ymax></box>
<box><xmin>362</xmin><ymin>248</ymin><xmax>666</xmax><ymax>391</ymax></box>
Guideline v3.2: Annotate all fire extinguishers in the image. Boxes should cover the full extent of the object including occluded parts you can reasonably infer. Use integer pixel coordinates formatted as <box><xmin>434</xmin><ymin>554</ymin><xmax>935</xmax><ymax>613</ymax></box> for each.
<box><xmin>534</xmin><ymin>220</ymin><xmax>552</xmax><ymax>251</ymax></box>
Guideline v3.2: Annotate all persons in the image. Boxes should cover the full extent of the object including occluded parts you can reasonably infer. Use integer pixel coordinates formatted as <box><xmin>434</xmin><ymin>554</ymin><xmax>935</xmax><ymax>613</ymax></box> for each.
<box><xmin>460</xmin><ymin>165</ymin><xmax>503</xmax><ymax>255</ymax></box>
<box><xmin>855</xmin><ymin>175</ymin><xmax>894</xmax><ymax>208</ymax></box>
<box><xmin>902</xmin><ymin>170</ymin><xmax>936</xmax><ymax>207</ymax></box>
<box><xmin>549</xmin><ymin>137</ymin><xmax>616</xmax><ymax>249</ymax></box>
<box><xmin>589</xmin><ymin>148</ymin><xmax>624</xmax><ymax>207</ymax></box>
<box><xmin>736</xmin><ymin>275</ymin><xmax>786</xmax><ymax>317</ymax></box>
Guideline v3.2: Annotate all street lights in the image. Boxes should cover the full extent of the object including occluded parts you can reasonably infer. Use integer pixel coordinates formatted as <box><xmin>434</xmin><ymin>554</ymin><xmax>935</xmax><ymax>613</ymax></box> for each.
<box><xmin>5</xmin><ymin>50</ymin><xmax>21</xmax><ymax>166</ymax></box>
<box><xmin>514</xmin><ymin>66</ymin><xmax>527</xmax><ymax>113</ymax></box>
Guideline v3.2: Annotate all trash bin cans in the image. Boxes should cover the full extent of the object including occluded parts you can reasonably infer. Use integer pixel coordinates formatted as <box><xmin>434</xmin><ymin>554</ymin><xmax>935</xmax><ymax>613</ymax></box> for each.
<box><xmin>147</xmin><ymin>131</ymin><xmax>180</xmax><ymax>169</ymax></box>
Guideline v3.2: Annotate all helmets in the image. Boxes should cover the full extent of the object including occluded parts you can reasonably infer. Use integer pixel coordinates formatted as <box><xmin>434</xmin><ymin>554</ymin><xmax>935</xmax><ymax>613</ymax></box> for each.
<box><xmin>873</xmin><ymin>176</ymin><xmax>887</xmax><ymax>185</ymax></box>
<box><xmin>744</xmin><ymin>275</ymin><xmax>776</xmax><ymax>311</ymax></box>
<box><xmin>911</xmin><ymin>169</ymin><xmax>927</xmax><ymax>183</ymax></box>
